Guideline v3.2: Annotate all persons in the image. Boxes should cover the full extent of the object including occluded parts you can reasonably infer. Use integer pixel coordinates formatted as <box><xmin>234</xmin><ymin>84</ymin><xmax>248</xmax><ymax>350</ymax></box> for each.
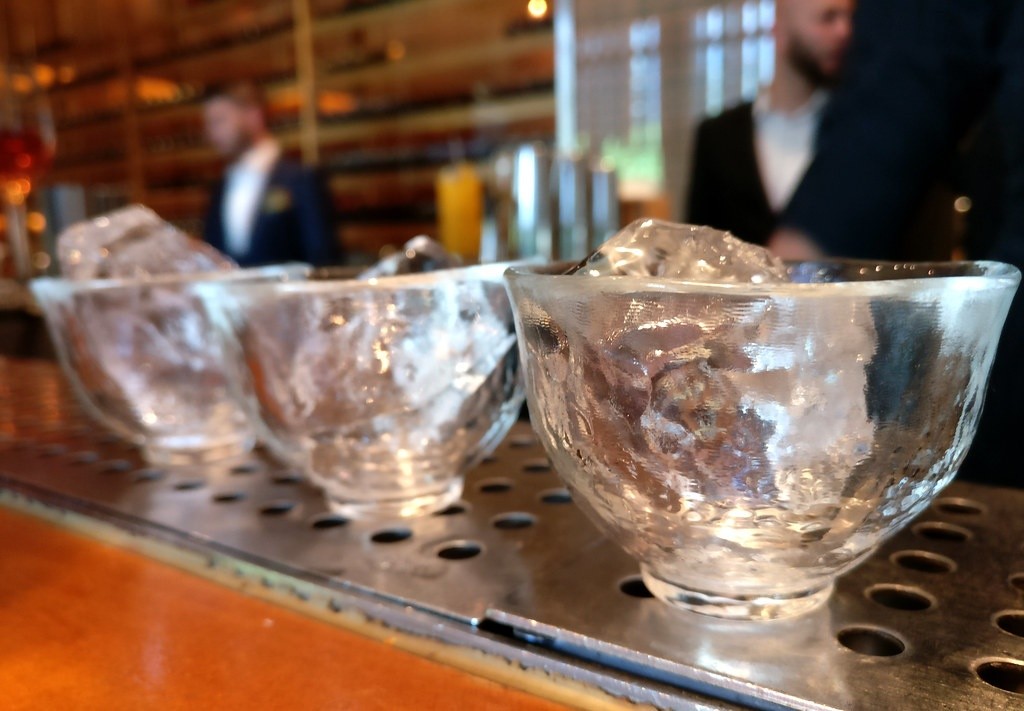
<box><xmin>201</xmin><ymin>72</ymin><xmax>344</xmax><ymax>267</ymax></box>
<box><xmin>682</xmin><ymin>1</ymin><xmax>924</xmax><ymax>267</ymax></box>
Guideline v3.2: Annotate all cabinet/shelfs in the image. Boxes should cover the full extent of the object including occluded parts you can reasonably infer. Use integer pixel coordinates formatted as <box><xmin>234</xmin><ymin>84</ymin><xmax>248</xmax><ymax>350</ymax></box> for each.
<box><xmin>0</xmin><ymin>0</ymin><xmax>557</xmax><ymax>269</ymax></box>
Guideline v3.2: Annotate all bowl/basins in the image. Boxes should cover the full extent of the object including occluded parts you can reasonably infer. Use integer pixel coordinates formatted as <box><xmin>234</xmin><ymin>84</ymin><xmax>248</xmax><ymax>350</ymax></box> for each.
<box><xmin>506</xmin><ymin>260</ymin><xmax>1023</xmax><ymax>625</ymax></box>
<box><xmin>198</xmin><ymin>260</ymin><xmax>577</xmax><ymax>519</ymax></box>
<box><xmin>30</xmin><ymin>266</ymin><xmax>314</xmax><ymax>467</ymax></box>
